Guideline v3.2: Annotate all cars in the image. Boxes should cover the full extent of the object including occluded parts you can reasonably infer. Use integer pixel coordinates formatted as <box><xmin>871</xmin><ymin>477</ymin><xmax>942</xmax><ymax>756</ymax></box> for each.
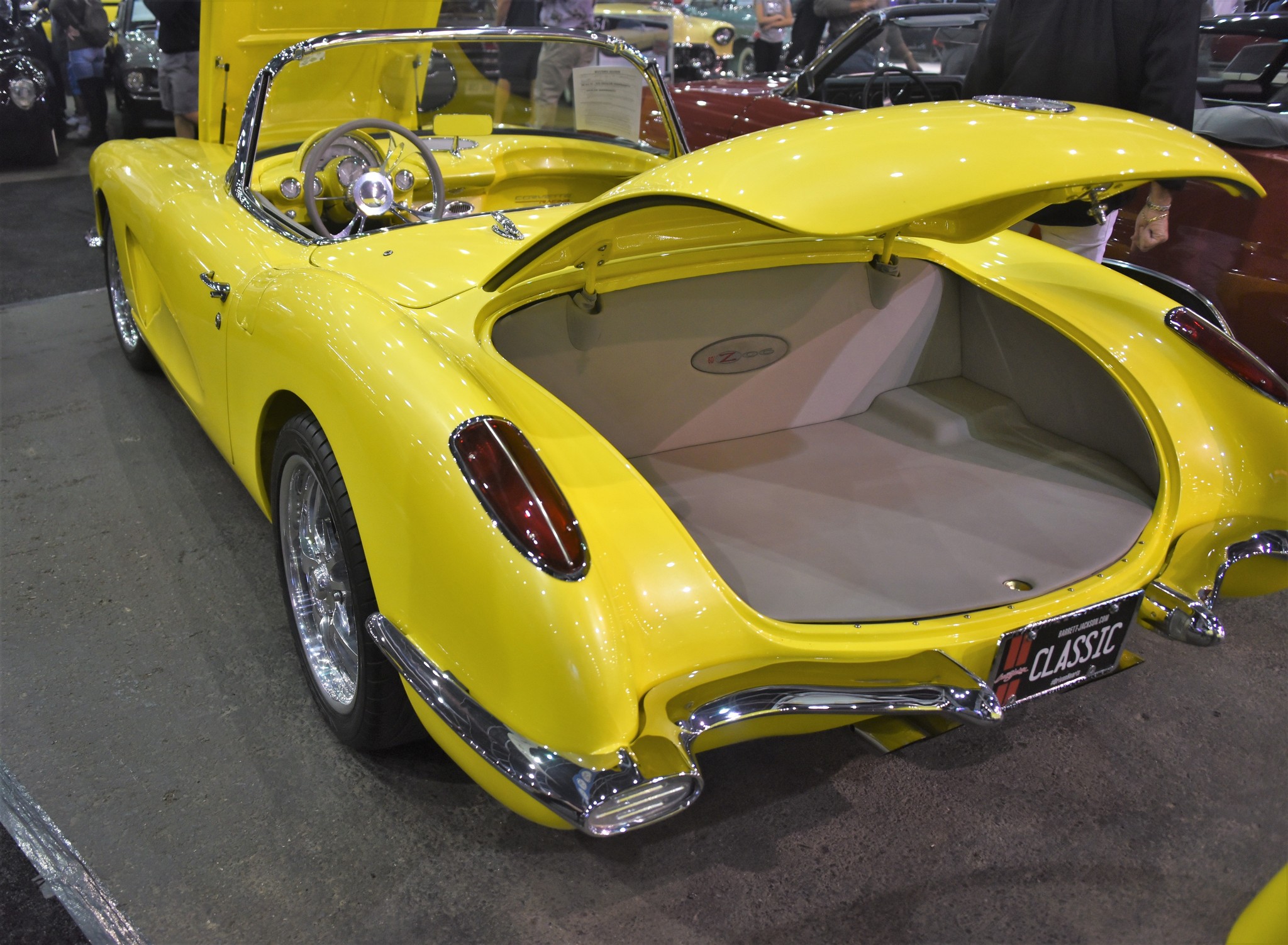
<box><xmin>1</xmin><ymin>0</ymin><xmax>174</xmax><ymax>166</ymax></box>
<box><xmin>446</xmin><ymin>0</ymin><xmax>1288</xmax><ymax>380</ymax></box>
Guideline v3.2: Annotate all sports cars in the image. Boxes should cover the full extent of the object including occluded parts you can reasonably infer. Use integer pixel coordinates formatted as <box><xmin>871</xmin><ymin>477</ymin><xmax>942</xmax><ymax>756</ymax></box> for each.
<box><xmin>87</xmin><ymin>1</ymin><xmax>1288</xmax><ymax>843</ymax></box>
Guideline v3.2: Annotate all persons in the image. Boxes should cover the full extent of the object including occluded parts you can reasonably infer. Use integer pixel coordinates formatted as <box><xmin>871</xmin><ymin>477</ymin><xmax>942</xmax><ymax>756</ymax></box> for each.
<box><xmin>963</xmin><ymin>0</ymin><xmax>1202</xmax><ymax>265</ymax></box>
<box><xmin>530</xmin><ymin>0</ymin><xmax>597</xmax><ymax>128</ymax></box>
<box><xmin>813</xmin><ymin>0</ymin><xmax>922</xmax><ymax>75</ymax></box>
<box><xmin>784</xmin><ymin>0</ymin><xmax>828</xmax><ymax>68</ymax></box>
<box><xmin>142</xmin><ymin>0</ymin><xmax>201</xmax><ymax>137</ymax></box>
<box><xmin>31</xmin><ymin>0</ymin><xmax>109</xmax><ymax>143</ymax></box>
<box><xmin>754</xmin><ymin>0</ymin><xmax>794</xmax><ymax>73</ymax></box>
<box><xmin>494</xmin><ymin>0</ymin><xmax>544</xmax><ymax>123</ymax></box>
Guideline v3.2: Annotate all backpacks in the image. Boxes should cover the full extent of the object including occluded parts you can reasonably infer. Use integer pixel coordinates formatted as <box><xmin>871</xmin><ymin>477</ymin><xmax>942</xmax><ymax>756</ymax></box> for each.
<box><xmin>69</xmin><ymin>0</ymin><xmax>109</xmax><ymax>48</ymax></box>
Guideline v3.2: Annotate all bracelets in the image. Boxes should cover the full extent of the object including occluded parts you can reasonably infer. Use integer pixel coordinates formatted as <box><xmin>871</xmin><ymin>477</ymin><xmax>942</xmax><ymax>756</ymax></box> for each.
<box><xmin>1146</xmin><ymin>197</ymin><xmax>1171</xmax><ymax>211</ymax></box>
<box><xmin>1142</xmin><ymin>207</ymin><xmax>1169</xmax><ymax>223</ymax></box>
<box><xmin>904</xmin><ymin>52</ymin><xmax>912</xmax><ymax>59</ymax></box>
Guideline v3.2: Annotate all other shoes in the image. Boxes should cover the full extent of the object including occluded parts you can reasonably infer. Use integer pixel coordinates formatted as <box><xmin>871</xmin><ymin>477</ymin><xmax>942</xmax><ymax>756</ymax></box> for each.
<box><xmin>67</xmin><ymin>115</ymin><xmax>80</xmax><ymax>125</ymax></box>
<box><xmin>67</xmin><ymin>129</ymin><xmax>88</xmax><ymax>139</ymax></box>
